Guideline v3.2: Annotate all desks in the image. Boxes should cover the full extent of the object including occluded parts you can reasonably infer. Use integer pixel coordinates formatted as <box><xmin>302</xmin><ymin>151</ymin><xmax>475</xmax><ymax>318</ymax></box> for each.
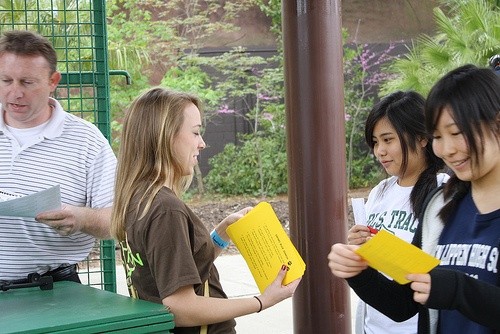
<box><xmin>0</xmin><ymin>280</ymin><xmax>175</xmax><ymax>334</ymax></box>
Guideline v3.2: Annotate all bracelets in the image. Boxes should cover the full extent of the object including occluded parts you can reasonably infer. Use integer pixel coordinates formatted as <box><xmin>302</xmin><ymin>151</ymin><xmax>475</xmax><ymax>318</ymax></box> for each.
<box><xmin>254</xmin><ymin>296</ymin><xmax>262</xmax><ymax>314</ymax></box>
<box><xmin>210</xmin><ymin>230</ymin><xmax>231</xmax><ymax>250</ymax></box>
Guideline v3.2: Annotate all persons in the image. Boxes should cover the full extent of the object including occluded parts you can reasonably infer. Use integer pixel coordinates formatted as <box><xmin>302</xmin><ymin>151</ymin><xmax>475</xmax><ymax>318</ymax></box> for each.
<box><xmin>0</xmin><ymin>30</ymin><xmax>118</xmax><ymax>286</ymax></box>
<box><xmin>327</xmin><ymin>64</ymin><xmax>500</xmax><ymax>334</ymax></box>
<box><xmin>111</xmin><ymin>89</ymin><xmax>302</xmax><ymax>333</ymax></box>
